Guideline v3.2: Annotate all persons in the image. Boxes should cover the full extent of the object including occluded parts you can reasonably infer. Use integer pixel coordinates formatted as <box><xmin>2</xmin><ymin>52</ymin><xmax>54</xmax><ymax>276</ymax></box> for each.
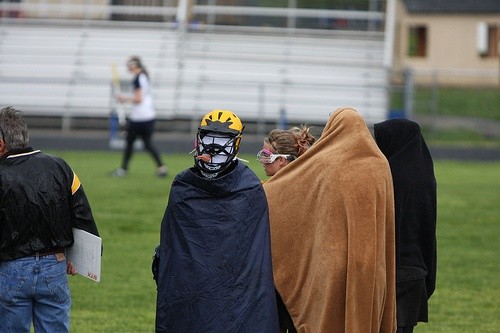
<box><xmin>108</xmin><ymin>57</ymin><xmax>168</xmax><ymax>178</ymax></box>
<box><xmin>152</xmin><ymin>109</ymin><xmax>280</xmax><ymax>333</ymax></box>
<box><xmin>0</xmin><ymin>107</ymin><xmax>103</xmax><ymax>333</ymax></box>
<box><xmin>258</xmin><ymin>107</ymin><xmax>437</xmax><ymax>333</ymax></box>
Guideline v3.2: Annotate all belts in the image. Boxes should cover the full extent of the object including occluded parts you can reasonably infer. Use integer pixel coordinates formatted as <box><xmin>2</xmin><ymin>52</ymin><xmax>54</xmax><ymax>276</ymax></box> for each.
<box><xmin>30</xmin><ymin>249</ymin><xmax>64</xmax><ymax>256</ymax></box>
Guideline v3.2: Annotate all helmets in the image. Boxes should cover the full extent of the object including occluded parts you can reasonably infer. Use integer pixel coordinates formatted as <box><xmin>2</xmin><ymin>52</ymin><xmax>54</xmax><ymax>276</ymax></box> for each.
<box><xmin>189</xmin><ymin>110</ymin><xmax>245</xmax><ymax>179</ymax></box>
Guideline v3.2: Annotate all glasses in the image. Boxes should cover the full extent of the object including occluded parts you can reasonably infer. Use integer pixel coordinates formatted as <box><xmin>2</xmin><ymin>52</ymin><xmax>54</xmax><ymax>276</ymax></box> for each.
<box><xmin>256</xmin><ymin>149</ymin><xmax>291</xmax><ymax>165</ymax></box>
